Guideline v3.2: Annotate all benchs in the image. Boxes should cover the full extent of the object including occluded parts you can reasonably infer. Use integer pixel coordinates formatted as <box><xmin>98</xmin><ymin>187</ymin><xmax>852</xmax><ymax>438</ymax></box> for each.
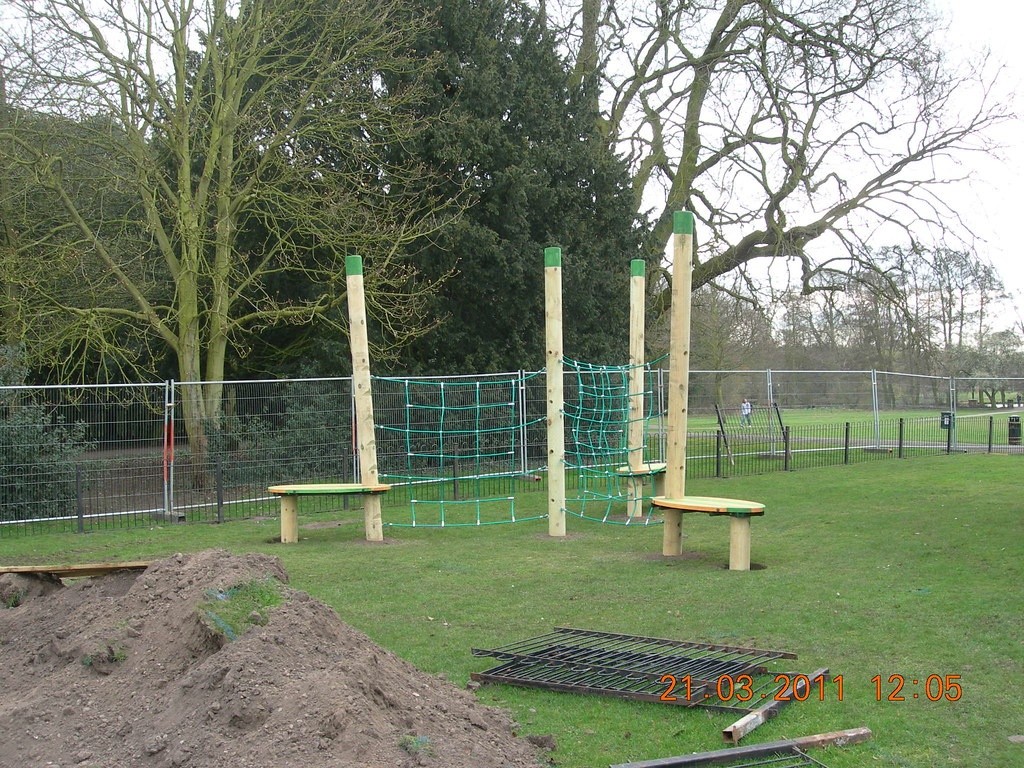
<box><xmin>266</xmin><ymin>483</ymin><xmax>390</xmax><ymax>546</ymax></box>
<box><xmin>648</xmin><ymin>497</ymin><xmax>766</xmax><ymax>570</ymax></box>
<box><xmin>614</xmin><ymin>462</ymin><xmax>668</xmax><ymax>518</ymax></box>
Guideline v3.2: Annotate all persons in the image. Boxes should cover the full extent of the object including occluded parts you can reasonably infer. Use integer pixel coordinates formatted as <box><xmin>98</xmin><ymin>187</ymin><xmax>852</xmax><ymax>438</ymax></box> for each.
<box><xmin>741</xmin><ymin>399</ymin><xmax>752</xmax><ymax>427</ymax></box>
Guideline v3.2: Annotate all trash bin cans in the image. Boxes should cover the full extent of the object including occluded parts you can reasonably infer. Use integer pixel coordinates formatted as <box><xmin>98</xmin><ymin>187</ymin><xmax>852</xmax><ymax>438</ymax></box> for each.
<box><xmin>1008</xmin><ymin>415</ymin><xmax>1022</xmax><ymax>445</ymax></box>
